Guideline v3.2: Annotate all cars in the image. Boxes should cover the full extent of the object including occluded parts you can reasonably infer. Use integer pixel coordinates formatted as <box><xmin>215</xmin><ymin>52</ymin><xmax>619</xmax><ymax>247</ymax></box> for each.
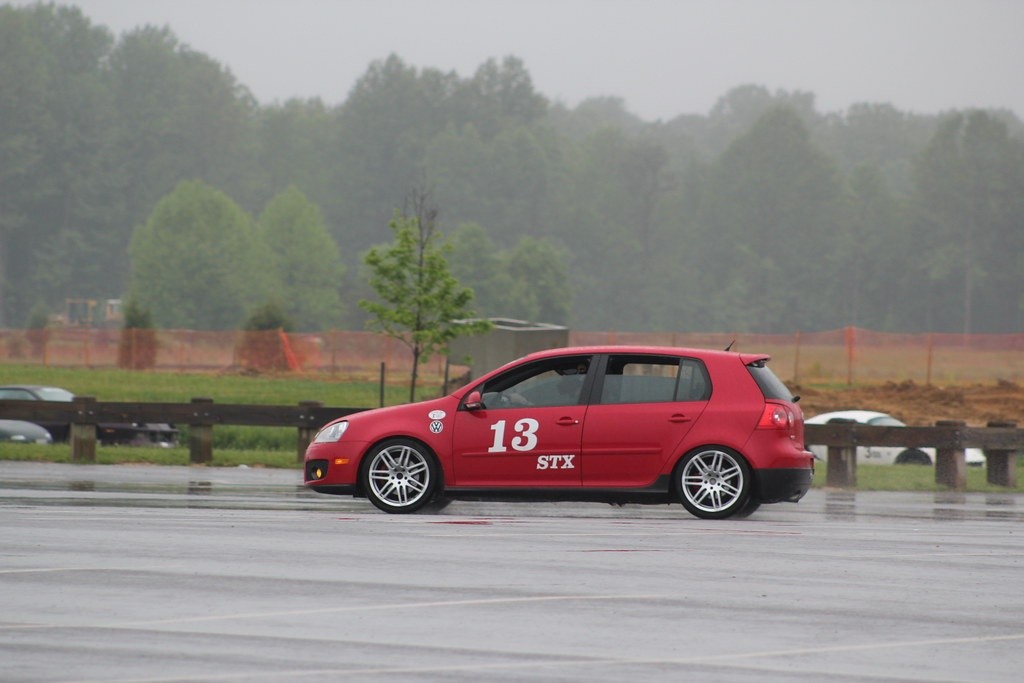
<box><xmin>803</xmin><ymin>409</ymin><xmax>987</xmax><ymax>469</ymax></box>
<box><xmin>302</xmin><ymin>344</ymin><xmax>814</xmax><ymax>520</ymax></box>
<box><xmin>0</xmin><ymin>384</ymin><xmax>178</xmax><ymax>448</ymax></box>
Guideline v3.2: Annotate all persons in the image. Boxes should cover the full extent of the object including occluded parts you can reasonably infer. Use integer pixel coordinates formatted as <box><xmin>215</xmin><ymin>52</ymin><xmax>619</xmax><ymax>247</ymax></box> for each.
<box><xmin>506</xmin><ymin>369</ymin><xmax>579</xmax><ymax>407</ymax></box>
